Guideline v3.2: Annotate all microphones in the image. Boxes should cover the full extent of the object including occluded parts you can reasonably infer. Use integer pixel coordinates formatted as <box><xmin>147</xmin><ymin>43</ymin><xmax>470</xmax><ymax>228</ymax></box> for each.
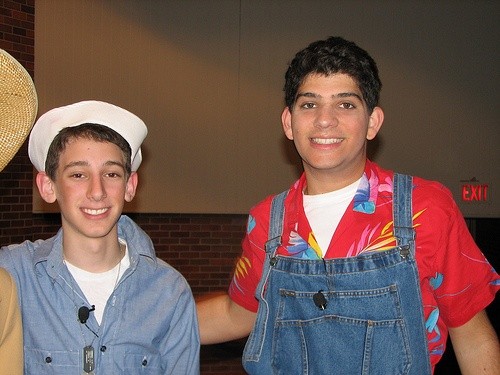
<box><xmin>311</xmin><ymin>290</ymin><xmax>328</xmax><ymax>311</ymax></box>
<box><xmin>77</xmin><ymin>306</ymin><xmax>95</xmax><ymax>324</ymax></box>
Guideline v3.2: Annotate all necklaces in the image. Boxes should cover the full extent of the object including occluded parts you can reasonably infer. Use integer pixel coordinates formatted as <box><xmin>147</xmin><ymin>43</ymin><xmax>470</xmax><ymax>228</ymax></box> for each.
<box><xmin>74</xmin><ymin>259</ymin><xmax>122</xmax><ymax>375</ymax></box>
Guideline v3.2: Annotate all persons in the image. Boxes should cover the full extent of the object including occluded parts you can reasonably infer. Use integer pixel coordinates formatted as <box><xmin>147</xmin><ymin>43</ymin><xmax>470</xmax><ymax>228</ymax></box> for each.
<box><xmin>194</xmin><ymin>36</ymin><xmax>500</xmax><ymax>375</ymax></box>
<box><xmin>0</xmin><ymin>101</ymin><xmax>199</xmax><ymax>375</ymax></box>
<box><xmin>0</xmin><ymin>267</ymin><xmax>23</xmax><ymax>375</ymax></box>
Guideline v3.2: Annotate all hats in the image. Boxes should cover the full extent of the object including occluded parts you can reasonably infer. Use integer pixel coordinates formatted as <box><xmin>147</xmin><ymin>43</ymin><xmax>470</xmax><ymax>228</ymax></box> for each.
<box><xmin>27</xmin><ymin>100</ymin><xmax>148</xmax><ymax>172</ymax></box>
<box><xmin>0</xmin><ymin>48</ymin><xmax>39</xmax><ymax>172</ymax></box>
